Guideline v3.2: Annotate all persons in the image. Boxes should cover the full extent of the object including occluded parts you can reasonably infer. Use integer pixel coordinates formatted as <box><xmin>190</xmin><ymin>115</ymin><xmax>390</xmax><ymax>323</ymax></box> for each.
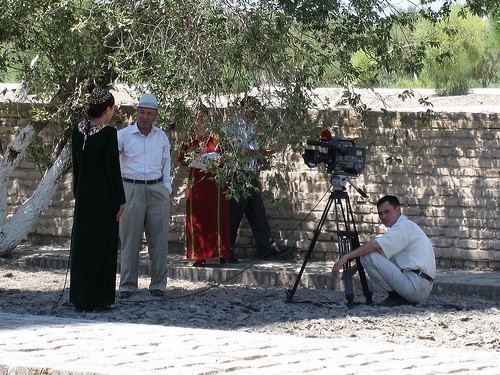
<box><xmin>178</xmin><ymin>107</ymin><xmax>231</xmax><ymax>266</ymax></box>
<box><xmin>117</xmin><ymin>93</ymin><xmax>172</xmax><ymax>298</ymax></box>
<box><xmin>224</xmin><ymin>96</ymin><xmax>291</xmax><ymax>261</ymax></box>
<box><xmin>333</xmin><ymin>195</ymin><xmax>436</xmax><ymax>306</ymax></box>
<box><xmin>69</xmin><ymin>89</ymin><xmax>126</xmax><ymax>311</ymax></box>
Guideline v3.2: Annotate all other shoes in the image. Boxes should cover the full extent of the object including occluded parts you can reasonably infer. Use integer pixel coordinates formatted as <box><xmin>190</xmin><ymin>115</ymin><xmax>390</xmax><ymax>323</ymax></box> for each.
<box><xmin>193</xmin><ymin>259</ymin><xmax>206</xmax><ymax>266</ymax></box>
<box><xmin>378</xmin><ymin>295</ymin><xmax>405</xmax><ymax>307</ymax></box>
<box><xmin>120</xmin><ymin>291</ymin><xmax>132</xmax><ymax>298</ymax></box>
<box><xmin>220</xmin><ymin>257</ymin><xmax>238</xmax><ymax>263</ymax></box>
<box><xmin>265</xmin><ymin>248</ymin><xmax>291</xmax><ymax>260</ymax></box>
<box><xmin>151</xmin><ymin>290</ymin><xmax>163</xmax><ymax>296</ymax></box>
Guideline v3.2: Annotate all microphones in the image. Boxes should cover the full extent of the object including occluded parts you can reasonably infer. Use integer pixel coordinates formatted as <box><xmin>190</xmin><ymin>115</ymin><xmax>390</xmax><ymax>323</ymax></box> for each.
<box><xmin>307</xmin><ymin>140</ymin><xmax>323</xmax><ymax>147</ymax></box>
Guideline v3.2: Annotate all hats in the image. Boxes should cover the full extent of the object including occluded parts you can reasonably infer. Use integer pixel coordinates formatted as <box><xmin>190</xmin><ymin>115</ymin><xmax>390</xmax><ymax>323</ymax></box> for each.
<box><xmin>87</xmin><ymin>87</ymin><xmax>110</xmax><ymax>105</ymax></box>
<box><xmin>138</xmin><ymin>93</ymin><xmax>158</xmax><ymax>108</ymax></box>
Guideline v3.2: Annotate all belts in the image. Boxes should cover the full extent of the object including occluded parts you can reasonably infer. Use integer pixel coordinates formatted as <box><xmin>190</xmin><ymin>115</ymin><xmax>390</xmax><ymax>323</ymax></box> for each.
<box><xmin>412</xmin><ymin>268</ymin><xmax>434</xmax><ymax>285</ymax></box>
<box><xmin>122</xmin><ymin>177</ymin><xmax>163</xmax><ymax>184</ymax></box>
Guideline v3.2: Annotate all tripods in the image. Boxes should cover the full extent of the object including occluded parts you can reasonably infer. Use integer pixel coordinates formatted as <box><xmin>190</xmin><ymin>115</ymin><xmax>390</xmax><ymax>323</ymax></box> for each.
<box><xmin>285</xmin><ymin>176</ymin><xmax>374</xmax><ymax>309</ymax></box>
